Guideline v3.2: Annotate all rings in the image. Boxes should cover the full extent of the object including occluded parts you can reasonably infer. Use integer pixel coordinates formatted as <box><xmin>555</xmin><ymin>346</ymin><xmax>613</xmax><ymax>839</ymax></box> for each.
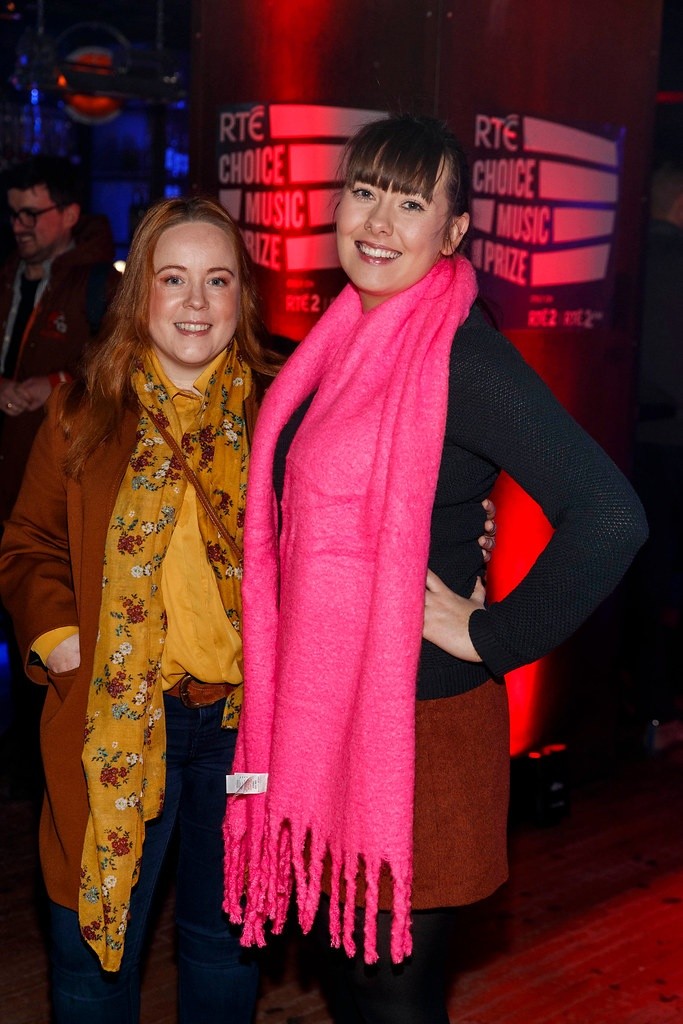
<box><xmin>6</xmin><ymin>401</ymin><xmax>14</xmax><ymax>409</ymax></box>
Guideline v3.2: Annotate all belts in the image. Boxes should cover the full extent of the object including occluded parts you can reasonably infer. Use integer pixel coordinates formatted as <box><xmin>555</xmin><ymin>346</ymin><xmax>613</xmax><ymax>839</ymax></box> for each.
<box><xmin>162</xmin><ymin>674</ymin><xmax>238</xmax><ymax>709</ymax></box>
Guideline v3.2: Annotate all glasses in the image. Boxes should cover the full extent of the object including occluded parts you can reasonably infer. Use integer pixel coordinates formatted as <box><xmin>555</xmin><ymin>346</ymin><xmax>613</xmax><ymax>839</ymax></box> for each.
<box><xmin>3</xmin><ymin>203</ymin><xmax>65</xmax><ymax>229</ymax></box>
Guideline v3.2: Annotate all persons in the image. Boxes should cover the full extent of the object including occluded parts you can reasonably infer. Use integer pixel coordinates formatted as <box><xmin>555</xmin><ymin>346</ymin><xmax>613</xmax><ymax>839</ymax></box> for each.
<box><xmin>1</xmin><ymin>199</ymin><xmax>496</xmax><ymax>1024</ymax></box>
<box><xmin>1</xmin><ymin>165</ymin><xmax>124</xmax><ymax>535</ymax></box>
<box><xmin>221</xmin><ymin>116</ymin><xmax>647</xmax><ymax>1024</ymax></box>
